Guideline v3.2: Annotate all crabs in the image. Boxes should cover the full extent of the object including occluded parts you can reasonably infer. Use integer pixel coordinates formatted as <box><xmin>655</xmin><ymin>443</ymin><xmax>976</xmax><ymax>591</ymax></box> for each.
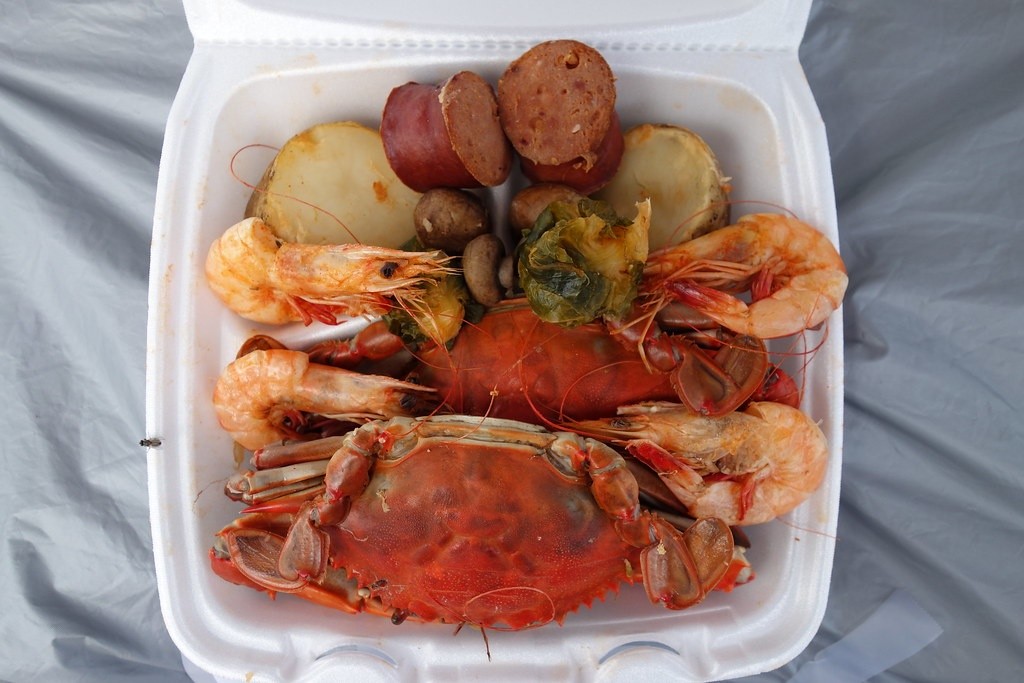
<box><xmin>210</xmin><ymin>411</ymin><xmax>757</xmax><ymax>664</ymax></box>
<box><xmin>237</xmin><ymin>285</ymin><xmax>771</xmax><ymax>444</ymax></box>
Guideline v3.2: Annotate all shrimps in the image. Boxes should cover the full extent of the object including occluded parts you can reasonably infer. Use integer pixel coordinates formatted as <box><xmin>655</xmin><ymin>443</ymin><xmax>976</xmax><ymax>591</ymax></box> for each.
<box><xmin>212</xmin><ymin>349</ymin><xmax>438</xmax><ymax>455</ymax></box>
<box><xmin>559</xmin><ymin>399</ymin><xmax>829</xmax><ymax>527</ymax></box>
<box><xmin>202</xmin><ymin>214</ymin><xmax>468</xmax><ymax>351</ymax></box>
<box><xmin>621</xmin><ymin>212</ymin><xmax>848</xmax><ymax>341</ymax></box>
